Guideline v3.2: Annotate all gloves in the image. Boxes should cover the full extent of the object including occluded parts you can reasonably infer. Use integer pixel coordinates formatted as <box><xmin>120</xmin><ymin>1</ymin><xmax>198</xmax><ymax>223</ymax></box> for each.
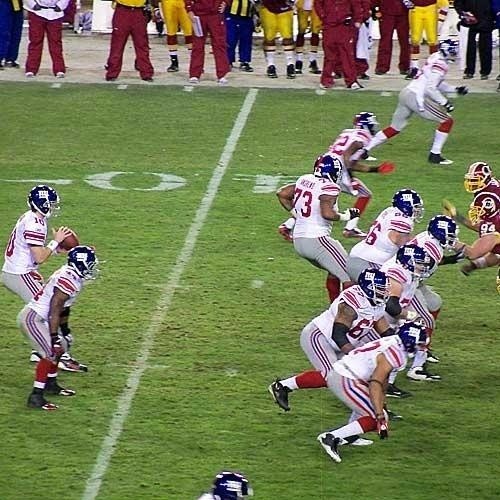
<box><xmin>442</xmin><ymin>252</ymin><xmax>465</xmax><ymax>264</ymax></box>
<box><xmin>402</xmin><ymin>0</ymin><xmax>415</xmax><ymax>10</ymax></box>
<box><xmin>377</xmin><ymin>417</ymin><xmax>389</xmax><ymax>440</ymax></box>
<box><xmin>371</xmin><ymin>4</ymin><xmax>382</xmax><ymax>22</ymax></box>
<box><xmin>457</xmin><ymin>9</ymin><xmax>480</xmax><ymax>28</ymax></box>
<box><xmin>50</xmin><ymin>334</ymin><xmax>64</xmax><ymax>354</ymax></box>
<box><xmin>63</xmin><ymin>329</ymin><xmax>75</xmax><ymax>344</ymax></box>
<box><xmin>150</xmin><ymin>6</ymin><xmax>164</xmax><ymax>24</ymax></box>
<box><xmin>343</xmin><ymin>207</ymin><xmax>361</xmax><ymax>222</ymax></box>
<box><xmin>456</xmin><ymin>83</ymin><xmax>471</xmax><ymax>95</ymax></box>
<box><xmin>460</xmin><ymin>260</ymin><xmax>477</xmax><ymax>276</ymax></box>
<box><xmin>440</xmin><ymin>199</ymin><xmax>458</xmax><ymax>220</ymax></box>
<box><xmin>384</xmin><ymin>295</ymin><xmax>402</xmax><ymax>318</ymax></box>
<box><xmin>406</xmin><ymin>310</ymin><xmax>425</xmax><ymax>326</ymax></box>
<box><xmin>376</xmin><ymin>158</ymin><xmax>395</xmax><ymax>174</ymax></box>
<box><xmin>444</xmin><ymin>100</ymin><xmax>456</xmax><ymax>114</ymax></box>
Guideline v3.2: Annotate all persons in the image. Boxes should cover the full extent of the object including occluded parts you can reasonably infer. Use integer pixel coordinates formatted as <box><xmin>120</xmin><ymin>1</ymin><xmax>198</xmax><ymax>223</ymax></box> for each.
<box><xmin>17</xmin><ymin>245</ymin><xmax>99</xmax><ymax>412</ymax></box>
<box><xmin>278</xmin><ymin>111</ymin><xmax>394</xmax><ymax>243</ymax></box>
<box><xmin>421</xmin><ymin>0</ymin><xmax>449</xmax><ymax>45</ymax></box>
<box><xmin>151</xmin><ymin>0</ymin><xmax>192</xmax><ymax>72</ymax></box>
<box><xmin>403</xmin><ymin>0</ymin><xmax>448</xmax><ymax>80</ymax></box>
<box><xmin>350</xmin><ymin>34</ymin><xmax>469</xmax><ymax>165</ymax></box>
<box><xmin>144</xmin><ymin>0</ymin><xmax>164</xmax><ymax>38</ymax></box>
<box><xmin>225</xmin><ymin>0</ymin><xmax>256</xmax><ymax>72</ymax></box>
<box><xmin>318</xmin><ymin>323</ymin><xmax>430</xmax><ymax>463</ymax></box>
<box><xmin>331</xmin><ymin>0</ymin><xmax>371</xmax><ymax>80</ymax></box>
<box><xmin>372</xmin><ymin>0</ymin><xmax>412</xmax><ymax>75</ymax></box>
<box><xmin>383</xmin><ymin>245</ymin><xmax>425</xmax><ymax>398</ymax></box>
<box><xmin>276</xmin><ymin>154</ymin><xmax>358</xmax><ymax>302</ymax></box>
<box><xmin>452</xmin><ymin>192</ymin><xmax>500</xmax><ymax>293</ymax></box>
<box><xmin>197</xmin><ymin>470</ymin><xmax>254</xmax><ymax>500</ymax></box>
<box><xmin>313</xmin><ymin>0</ymin><xmax>362</xmax><ymax>95</ymax></box>
<box><xmin>0</xmin><ymin>0</ymin><xmax>24</xmax><ymax>70</ymax></box>
<box><xmin>294</xmin><ymin>0</ymin><xmax>323</xmax><ymax>73</ymax></box>
<box><xmin>442</xmin><ymin>162</ymin><xmax>500</xmax><ymax>232</ymax></box>
<box><xmin>453</xmin><ymin>0</ymin><xmax>493</xmax><ymax>80</ymax></box>
<box><xmin>268</xmin><ymin>268</ymin><xmax>404</xmax><ymax>421</ymax></box>
<box><xmin>104</xmin><ymin>0</ymin><xmax>154</xmax><ymax>82</ymax></box>
<box><xmin>23</xmin><ymin>0</ymin><xmax>70</xmax><ymax>78</ymax></box>
<box><xmin>381</xmin><ymin>214</ymin><xmax>465</xmax><ymax>381</ymax></box>
<box><xmin>493</xmin><ymin>0</ymin><xmax>500</xmax><ymax>92</ymax></box>
<box><xmin>184</xmin><ymin>0</ymin><xmax>231</xmax><ymax>83</ymax></box>
<box><xmin>3</xmin><ymin>185</ymin><xmax>73</xmax><ymax>362</ymax></box>
<box><xmin>254</xmin><ymin>0</ymin><xmax>297</xmax><ymax>79</ymax></box>
<box><xmin>345</xmin><ymin>189</ymin><xmax>424</xmax><ymax>283</ymax></box>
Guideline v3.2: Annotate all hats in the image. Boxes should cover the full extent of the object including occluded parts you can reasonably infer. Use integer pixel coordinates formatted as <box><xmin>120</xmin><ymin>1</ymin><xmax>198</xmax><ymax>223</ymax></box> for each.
<box><xmin>356</xmin><ymin>266</ymin><xmax>392</xmax><ymax>306</ymax></box>
<box><xmin>467</xmin><ymin>190</ymin><xmax>500</xmax><ymax>227</ymax></box>
<box><xmin>25</xmin><ymin>183</ymin><xmax>62</xmax><ymax>219</ymax></box>
<box><xmin>392</xmin><ymin>186</ymin><xmax>425</xmax><ymax>223</ymax></box>
<box><xmin>462</xmin><ymin>160</ymin><xmax>494</xmax><ymax>194</ymax></box>
<box><xmin>394</xmin><ymin>242</ymin><xmax>432</xmax><ymax>282</ymax></box>
<box><xmin>425</xmin><ymin>214</ymin><xmax>461</xmax><ymax>253</ymax></box>
<box><xmin>66</xmin><ymin>243</ymin><xmax>100</xmax><ymax>281</ymax></box>
<box><xmin>312</xmin><ymin>153</ymin><xmax>344</xmax><ymax>186</ymax></box>
<box><xmin>352</xmin><ymin>109</ymin><xmax>381</xmax><ymax>135</ymax></box>
<box><xmin>211</xmin><ymin>470</ymin><xmax>253</xmax><ymax>500</ymax></box>
<box><xmin>397</xmin><ymin>321</ymin><xmax>428</xmax><ymax>357</ymax></box>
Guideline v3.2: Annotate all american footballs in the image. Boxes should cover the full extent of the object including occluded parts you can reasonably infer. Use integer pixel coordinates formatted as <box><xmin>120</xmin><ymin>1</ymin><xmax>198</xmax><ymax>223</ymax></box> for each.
<box><xmin>56</xmin><ymin>228</ymin><xmax>80</xmax><ymax>251</ymax></box>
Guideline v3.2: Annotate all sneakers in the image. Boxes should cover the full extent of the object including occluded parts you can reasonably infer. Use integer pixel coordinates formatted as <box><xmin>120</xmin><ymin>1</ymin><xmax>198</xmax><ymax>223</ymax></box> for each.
<box><xmin>386</xmin><ymin>409</ymin><xmax>404</xmax><ymax>424</ymax></box>
<box><xmin>47</xmin><ymin>383</ymin><xmax>76</xmax><ymax>397</ymax></box>
<box><xmin>307</xmin><ymin>66</ymin><xmax>321</xmax><ymax>74</ymax></box>
<box><xmin>266</xmin><ymin>68</ymin><xmax>277</xmax><ymax>78</ymax></box>
<box><xmin>427</xmin><ymin>152</ymin><xmax>454</xmax><ymax>166</ymax></box>
<box><xmin>318</xmin><ymin>82</ymin><xmax>330</xmax><ymax>89</ymax></box>
<box><xmin>298</xmin><ymin>67</ymin><xmax>303</xmax><ymax>75</ymax></box>
<box><xmin>57</xmin><ymin>355</ymin><xmax>89</xmax><ymax>373</ymax></box>
<box><xmin>285</xmin><ymin>69</ymin><xmax>296</xmax><ymax>78</ymax></box>
<box><xmin>342</xmin><ymin>227</ymin><xmax>368</xmax><ymax>239</ymax></box>
<box><xmin>480</xmin><ymin>74</ymin><xmax>488</xmax><ymax>81</ymax></box>
<box><xmin>360</xmin><ymin>149</ymin><xmax>378</xmax><ymax>163</ymax></box>
<box><xmin>384</xmin><ymin>383</ymin><xmax>409</xmax><ymax>400</ymax></box>
<box><xmin>277</xmin><ymin>221</ymin><xmax>294</xmax><ymax>242</ymax></box>
<box><xmin>24</xmin><ymin>71</ymin><xmax>36</xmax><ymax>79</ymax></box>
<box><xmin>340</xmin><ymin>435</ymin><xmax>375</xmax><ymax>447</ymax></box>
<box><xmin>267</xmin><ymin>380</ymin><xmax>292</xmax><ymax>413</ymax></box>
<box><xmin>216</xmin><ymin>76</ymin><xmax>229</xmax><ymax>83</ymax></box>
<box><xmin>167</xmin><ymin>63</ymin><xmax>181</xmax><ymax>74</ymax></box>
<box><xmin>316</xmin><ymin>431</ymin><xmax>343</xmax><ymax>465</ymax></box>
<box><xmin>30</xmin><ymin>348</ymin><xmax>42</xmax><ymax>364</ymax></box>
<box><xmin>359</xmin><ymin>72</ymin><xmax>371</xmax><ymax>81</ymax></box>
<box><xmin>189</xmin><ymin>76</ymin><xmax>200</xmax><ymax>84</ymax></box>
<box><xmin>463</xmin><ymin>73</ymin><xmax>474</xmax><ymax>79</ymax></box>
<box><xmin>408</xmin><ymin>350</ymin><xmax>439</xmax><ymax>364</ymax></box>
<box><xmin>239</xmin><ymin>62</ymin><xmax>254</xmax><ymax>72</ymax></box>
<box><xmin>56</xmin><ymin>71</ymin><xmax>66</xmax><ymax>80</ymax></box>
<box><xmin>1</xmin><ymin>59</ymin><xmax>20</xmax><ymax>68</ymax></box>
<box><xmin>406</xmin><ymin>365</ymin><xmax>442</xmax><ymax>382</ymax></box>
<box><xmin>405</xmin><ymin>68</ymin><xmax>417</xmax><ymax>80</ymax></box>
<box><xmin>437</xmin><ymin>35</ymin><xmax>461</xmax><ymax>64</ymax></box>
<box><xmin>26</xmin><ymin>390</ymin><xmax>60</xmax><ymax>410</ymax></box>
<box><xmin>350</xmin><ymin>81</ymin><xmax>361</xmax><ymax>90</ymax></box>
